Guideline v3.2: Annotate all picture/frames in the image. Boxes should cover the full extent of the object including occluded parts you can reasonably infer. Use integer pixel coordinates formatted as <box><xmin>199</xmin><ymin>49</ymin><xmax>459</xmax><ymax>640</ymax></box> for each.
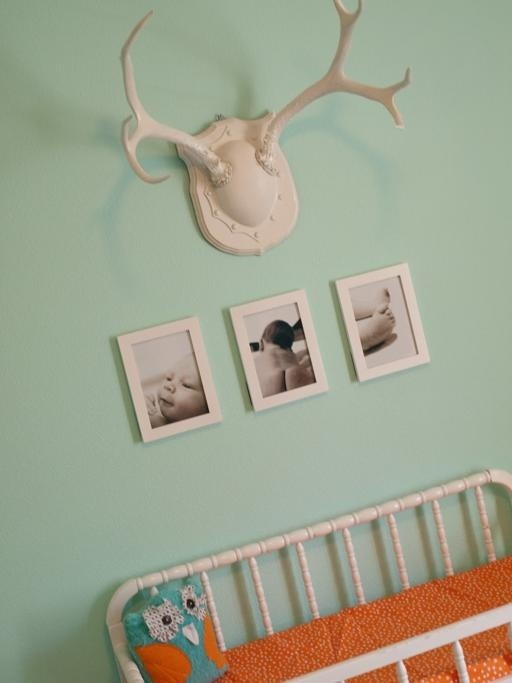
<box><xmin>228</xmin><ymin>288</ymin><xmax>330</xmax><ymax>413</ymax></box>
<box><xmin>115</xmin><ymin>314</ymin><xmax>225</xmax><ymax>444</ymax></box>
<box><xmin>334</xmin><ymin>261</ymin><xmax>431</xmax><ymax>383</ymax></box>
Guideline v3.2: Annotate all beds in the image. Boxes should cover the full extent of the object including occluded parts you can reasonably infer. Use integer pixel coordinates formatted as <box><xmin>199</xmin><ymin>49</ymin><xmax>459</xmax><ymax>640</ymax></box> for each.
<box><xmin>105</xmin><ymin>467</ymin><xmax>512</xmax><ymax>683</ymax></box>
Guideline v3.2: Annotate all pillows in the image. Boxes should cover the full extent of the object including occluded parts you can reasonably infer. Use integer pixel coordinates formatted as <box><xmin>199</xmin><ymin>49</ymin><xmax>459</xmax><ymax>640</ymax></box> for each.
<box><xmin>123</xmin><ymin>571</ymin><xmax>231</xmax><ymax>683</ymax></box>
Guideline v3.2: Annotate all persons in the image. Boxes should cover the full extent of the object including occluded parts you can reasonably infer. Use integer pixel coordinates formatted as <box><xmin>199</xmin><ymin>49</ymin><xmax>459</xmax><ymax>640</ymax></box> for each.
<box><xmin>248</xmin><ymin>315</ymin><xmax>314</xmax><ymax>398</ymax></box>
<box><xmin>354</xmin><ymin>286</ymin><xmax>397</xmax><ymax>352</ymax></box>
<box><xmin>134</xmin><ymin>346</ymin><xmax>207</xmax><ymax>433</ymax></box>
